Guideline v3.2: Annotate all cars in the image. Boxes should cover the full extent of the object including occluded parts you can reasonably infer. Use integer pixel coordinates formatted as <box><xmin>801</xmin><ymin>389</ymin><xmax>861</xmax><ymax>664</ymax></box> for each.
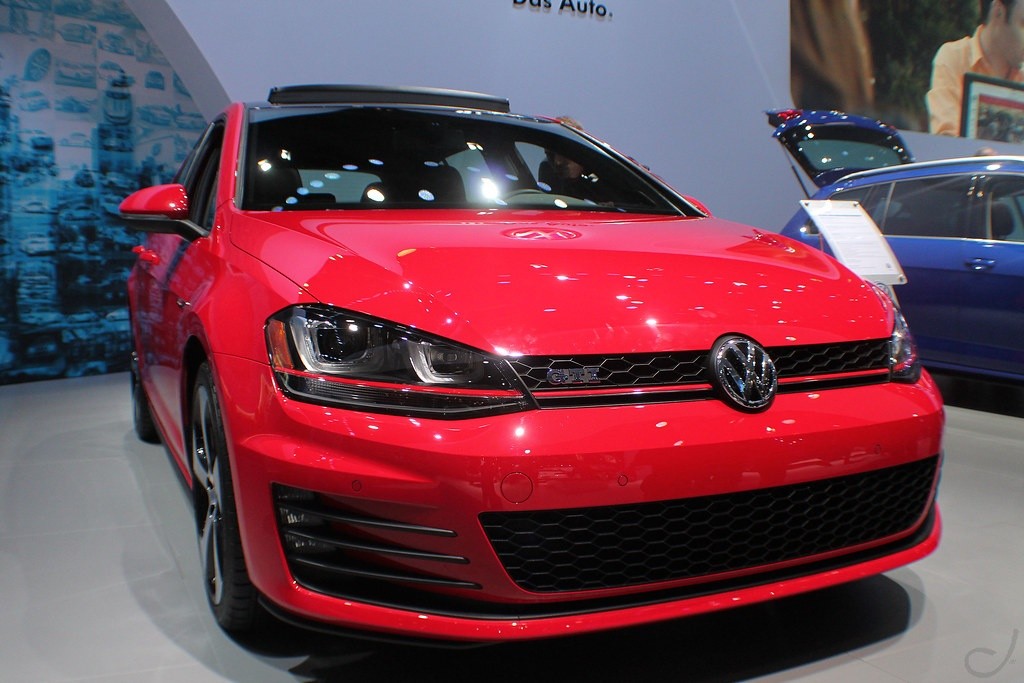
<box><xmin>116</xmin><ymin>83</ymin><xmax>946</xmax><ymax>644</ymax></box>
<box><xmin>764</xmin><ymin>107</ymin><xmax>1024</xmax><ymax>381</ymax></box>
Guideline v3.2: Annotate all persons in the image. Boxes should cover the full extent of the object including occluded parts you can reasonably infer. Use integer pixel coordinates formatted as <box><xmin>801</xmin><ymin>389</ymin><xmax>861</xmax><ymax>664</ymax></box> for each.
<box><xmin>925</xmin><ymin>0</ymin><xmax>1024</xmax><ymax>138</ymax></box>
<box><xmin>539</xmin><ymin>115</ymin><xmax>607</xmax><ymax>203</ymax></box>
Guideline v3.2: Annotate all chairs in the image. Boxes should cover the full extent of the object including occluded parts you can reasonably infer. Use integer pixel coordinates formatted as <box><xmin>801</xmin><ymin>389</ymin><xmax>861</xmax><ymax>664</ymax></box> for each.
<box><xmin>384</xmin><ymin>165</ymin><xmax>465</xmax><ymax>204</ymax></box>
<box><xmin>242</xmin><ymin>145</ymin><xmax>302</xmax><ymax>206</ymax></box>
